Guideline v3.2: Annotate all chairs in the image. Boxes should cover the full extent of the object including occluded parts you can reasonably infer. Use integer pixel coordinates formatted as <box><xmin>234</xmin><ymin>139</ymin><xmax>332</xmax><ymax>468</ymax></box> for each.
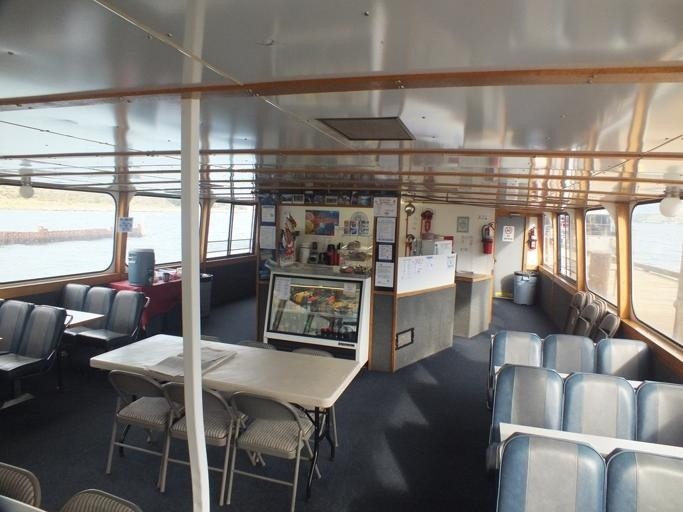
<box><xmin>636</xmin><ymin>381</ymin><xmax>683</xmax><ymax>447</ymax></box>
<box><xmin>0</xmin><ymin>305</ymin><xmax>73</xmax><ymax>410</ymax></box>
<box><xmin>544</xmin><ymin>334</ymin><xmax>598</xmax><ymax>380</ymax></box>
<box><xmin>562</xmin><ymin>291</ymin><xmax>621</xmax><ymax>343</ymax></box>
<box><xmin>225</xmin><ymin>390</ymin><xmax>321</xmax><ymax>512</ymax></box>
<box><xmin>105</xmin><ymin>370</ymin><xmax>185</xmax><ymax>489</ymax></box>
<box><xmin>292</xmin><ymin>349</ymin><xmax>340</xmax><ymax>448</ymax></box>
<box><xmin>496</xmin><ymin>435</ymin><xmax>607</xmax><ymax>512</ymax></box>
<box><xmin>160</xmin><ymin>381</ymin><xmax>256</xmax><ymax>507</ymax></box>
<box><xmin>565</xmin><ymin>372</ymin><xmax>637</xmax><ymax>443</ymax></box>
<box><xmin>0</xmin><ymin>461</ymin><xmax>42</xmax><ymax>507</ymax></box>
<box><xmin>485</xmin><ymin>363</ymin><xmax>564</xmax><ymax>471</ymax></box>
<box><xmin>77</xmin><ymin>290</ymin><xmax>151</xmax><ymax>352</ymax></box>
<box><xmin>597</xmin><ymin>339</ymin><xmax>651</xmax><ymax>380</ymax></box>
<box><xmin>606</xmin><ymin>450</ymin><xmax>683</xmax><ymax>512</ymax></box>
<box><xmin>57</xmin><ymin>283</ymin><xmax>90</xmax><ymax>311</ymax></box>
<box><xmin>58</xmin><ymin>489</ymin><xmax>140</xmax><ymax>512</ymax></box>
<box><xmin>486</xmin><ymin>330</ymin><xmax>543</xmax><ymax>410</ymax></box>
<box><xmin>64</xmin><ymin>286</ymin><xmax>116</xmax><ymax>345</ymax></box>
<box><xmin>0</xmin><ymin>300</ymin><xmax>35</xmax><ymax>356</ymax></box>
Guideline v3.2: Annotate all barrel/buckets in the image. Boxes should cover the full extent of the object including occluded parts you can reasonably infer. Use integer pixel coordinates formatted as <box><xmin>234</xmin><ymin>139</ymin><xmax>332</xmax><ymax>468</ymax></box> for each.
<box><xmin>128</xmin><ymin>248</ymin><xmax>155</xmax><ymax>286</ymax></box>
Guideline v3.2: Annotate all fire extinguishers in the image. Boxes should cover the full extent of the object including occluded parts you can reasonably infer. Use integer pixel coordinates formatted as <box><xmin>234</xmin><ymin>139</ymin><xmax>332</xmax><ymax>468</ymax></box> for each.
<box><xmin>481</xmin><ymin>222</ymin><xmax>495</xmax><ymax>254</ymax></box>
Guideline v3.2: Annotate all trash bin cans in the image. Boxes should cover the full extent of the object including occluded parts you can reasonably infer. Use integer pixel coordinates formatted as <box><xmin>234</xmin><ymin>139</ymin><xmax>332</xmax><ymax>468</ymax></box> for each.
<box><xmin>513</xmin><ymin>271</ymin><xmax>539</xmax><ymax>306</ymax></box>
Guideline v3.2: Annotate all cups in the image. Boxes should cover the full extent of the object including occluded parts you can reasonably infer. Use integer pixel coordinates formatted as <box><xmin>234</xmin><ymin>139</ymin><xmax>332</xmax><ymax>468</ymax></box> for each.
<box><xmin>162</xmin><ymin>273</ymin><xmax>170</xmax><ymax>282</ymax></box>
<box><xmin>169</xmin><ymin>274</ymin><xmax>174</xmax><ymax>279</ymax></box>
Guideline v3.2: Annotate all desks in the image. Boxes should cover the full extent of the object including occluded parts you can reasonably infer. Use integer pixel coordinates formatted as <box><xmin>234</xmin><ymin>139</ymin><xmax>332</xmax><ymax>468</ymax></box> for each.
<box><xmin>455</xmin><ymin>272</ymin><xmax>492</xmax><ymax>338</ymax></box>
<box><xmin>109</xmin><ymin>276</ymin><xmax>182</xmax><ymax>336</ymax></box>
<box><xmin>369</xmin><ymin>284</ymin><xmax>456</xmax><ymax>376</ymax></box>
<box><xmin>90</xmin><ymin>334</ymin><xmax>361</xmax><ymax>501</ymax></box>
<box><xmin>63</xmin><ymin>308</ymin><xmax>105</xmax><ymax>329</ymax></box>
<box><xmin>496</xmin><ymin>422</ymin><xmax>683</xmax><ymax>468</ymax></box>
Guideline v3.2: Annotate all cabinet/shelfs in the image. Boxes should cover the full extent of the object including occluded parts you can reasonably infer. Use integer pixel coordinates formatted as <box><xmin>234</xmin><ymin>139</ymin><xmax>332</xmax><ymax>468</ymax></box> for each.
<box><xmin>263</xmin><ymin>266</ymin><xmax>371</xmax><ymax>370</ymax></box>
